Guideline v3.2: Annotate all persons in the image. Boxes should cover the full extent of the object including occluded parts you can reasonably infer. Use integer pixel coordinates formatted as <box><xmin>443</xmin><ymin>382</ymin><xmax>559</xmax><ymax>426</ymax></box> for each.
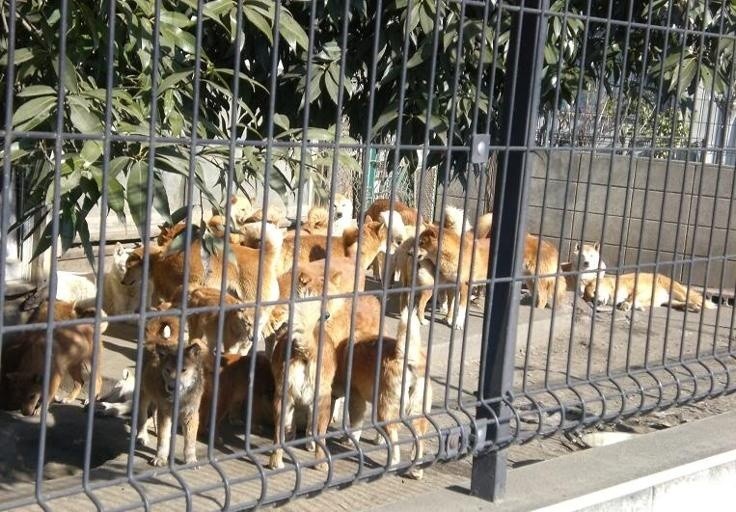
<box><xmin>560</xmin><ymin>140</ymin><xmax>570</xmax><ymax>147</ymax></box>
<box><xmin>546</xmin><ymin>131</ymin><xmax>559</xmax><ymax>149</ymax></box>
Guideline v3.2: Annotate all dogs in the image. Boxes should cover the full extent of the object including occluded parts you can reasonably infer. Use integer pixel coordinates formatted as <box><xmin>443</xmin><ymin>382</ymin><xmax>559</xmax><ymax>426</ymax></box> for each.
<box><xmin>572</xmin><ymin>240</ymin><xmax>606</xmax><ymax>297</ymax></box>
<box><xmin>4</xmin><ymin>185</ymin><xmax>567</xmax><ymax>480</ymax></box>
<box><xmin>581</xmin><ymin>271</ymin><xmax>719</xmax><ymax>312</ymax></box>
